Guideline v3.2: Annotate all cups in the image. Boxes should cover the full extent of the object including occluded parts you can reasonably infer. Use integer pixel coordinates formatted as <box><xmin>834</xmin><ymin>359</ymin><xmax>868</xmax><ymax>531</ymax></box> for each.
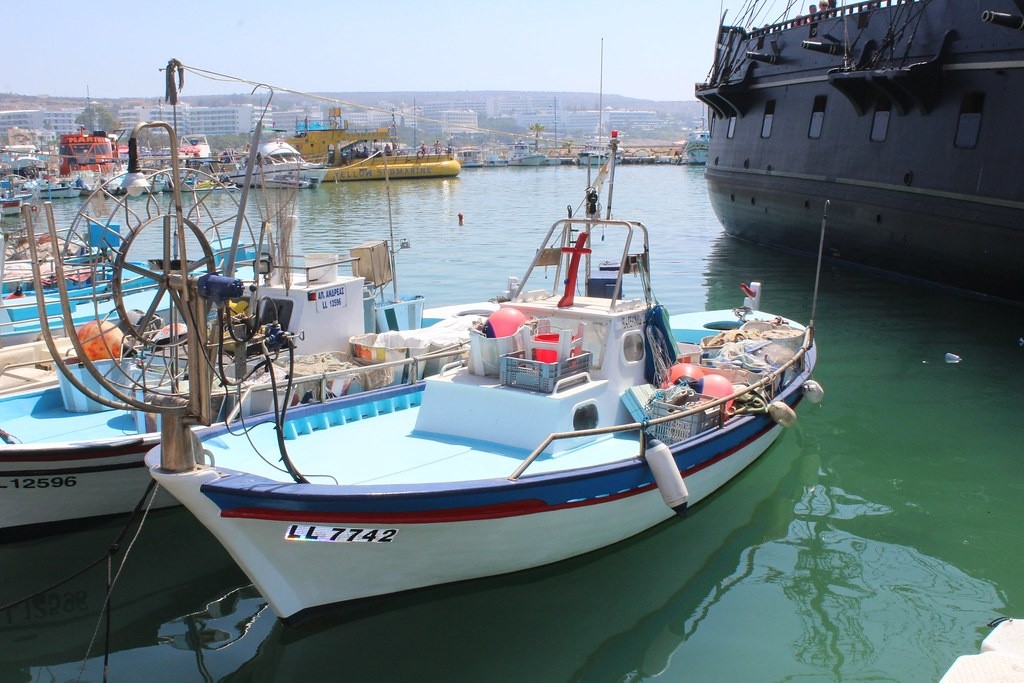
<box><xmin>945</xmin><ymin>353</ymin><xmax>959</xmax><ymax>363</ymax></box>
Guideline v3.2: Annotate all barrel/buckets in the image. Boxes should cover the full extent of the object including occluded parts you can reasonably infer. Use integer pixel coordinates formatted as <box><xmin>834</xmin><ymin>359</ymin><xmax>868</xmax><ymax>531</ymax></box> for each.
<box><xmin>536</xmin><ymin>334</ymin><xmax>574</xmax><ymax>363</ymax></box>
<box><xmin>677</xmin><ymin>342</ymin><xmax>701</xmax><ymax>365</ymax></box>
<box><xmin>364</xmin><ymin>281</ymin><xmax>377</xmax><ymax>333</ymax></box>
<box><xmin>304</xmin><ymin>253</ymin><xmax>338</xmax><ymax>284</ymax></box>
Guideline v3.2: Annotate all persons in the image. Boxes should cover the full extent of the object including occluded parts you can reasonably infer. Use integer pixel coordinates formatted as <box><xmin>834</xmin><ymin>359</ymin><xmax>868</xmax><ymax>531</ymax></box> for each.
<box><xmin>354</xmin><ymin>146</ymin><xmax>369</xmax><ymax>158</ymax></box>
<box><xmin>372</xmin><ymin>148</ymin><xmax>381</xmax><ymax>157</ymax></box>
<box><xmin>385</xmin><ymin>144</ymin><xmax>392</xmax><ymax>156</ymax></box>
<box><xmin>433</xmin><ymin>139</ymin><xmax>441</xmax><ymax>154</ymax></box>
<box><xmin>221</xmin><ymin>152</ymin><xmax>231</xmax><ymax>173</ymax></box>
<box><xmin>448</xmin><ymin>144</ymin><xmax>452</xmax><ymax>152</ymax></box>
<box><xmin>420</xmin><ymin>144</ymin><xmax>427</xmax><ymax>154</ymax></box>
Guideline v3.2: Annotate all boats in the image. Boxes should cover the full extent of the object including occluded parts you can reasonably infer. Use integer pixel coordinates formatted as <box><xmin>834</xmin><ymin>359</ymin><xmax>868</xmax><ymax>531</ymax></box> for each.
<box><xmin>685</xmin><ymin>102</ymin><xmax>709</xmax><ymax>162</ymax></box>
<box><xmin>696</xmin><ymin>0</ymin><xmax>1024</xmax><ymax>261</ymax></box>
<box><xmin>456</xmin><ymin>142</ymin><xmax>688</xmax><ymax>166</ymax></box>
<box><xmin>0</xmin><ymin>141</ymin><xmax>329</xmax><ymax>214</ymax></box>
<box><xmin>285</xmin><ymin>129</ymin><xmax>461</xmax><ymax>181</ymax></box>
<box><xmin>0</xmin><ymin>128</ymin><xmax>620</xmax><ymax>528</ymax></box>
<box><xmin>144</xmin><ymin>58</ymin><xmax>832</xmax><ymax>616</ymax></box>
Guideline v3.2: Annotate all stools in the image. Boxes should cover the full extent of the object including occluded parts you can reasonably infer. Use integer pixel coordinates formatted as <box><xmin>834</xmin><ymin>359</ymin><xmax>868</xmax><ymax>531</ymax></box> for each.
<box><xmin>523</xmin><ymin>319</ymin><xmax>588</xmax><ymax>369</ymax></box>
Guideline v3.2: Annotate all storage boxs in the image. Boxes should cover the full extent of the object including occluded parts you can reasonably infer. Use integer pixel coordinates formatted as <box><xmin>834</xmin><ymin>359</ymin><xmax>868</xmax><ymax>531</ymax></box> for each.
<box><xmin>499</xmin><ymin>349</ymin><xmax>593</xmax><ymax>392</ymax></box>
<box><xmin>376</xmin><ymin>297</ymin><xmax>427</xmax><ymax>336</ymax></box>
<box><xmin>621</xmin><ymin>383</ymin><xmax>656</xmax><ymax>428</ymax></box>
<box><xmin>468</xmin><ymin>316</ymin><xmax>538</xmax><ymax>381</ymax></box>
<box><xmin>651</xmin><ymin>393</ymin><xmax>728</xmax><ymax>444</ymax></box>
<box><xmin>350</xmin><ymin>240</ymin><xmax>391</xmax><ymax>288</ymax></box>
<box><xmin>57</xmin><ymin>358</ymin><xmax>133</xmax><ymax>411</ymax></box>
